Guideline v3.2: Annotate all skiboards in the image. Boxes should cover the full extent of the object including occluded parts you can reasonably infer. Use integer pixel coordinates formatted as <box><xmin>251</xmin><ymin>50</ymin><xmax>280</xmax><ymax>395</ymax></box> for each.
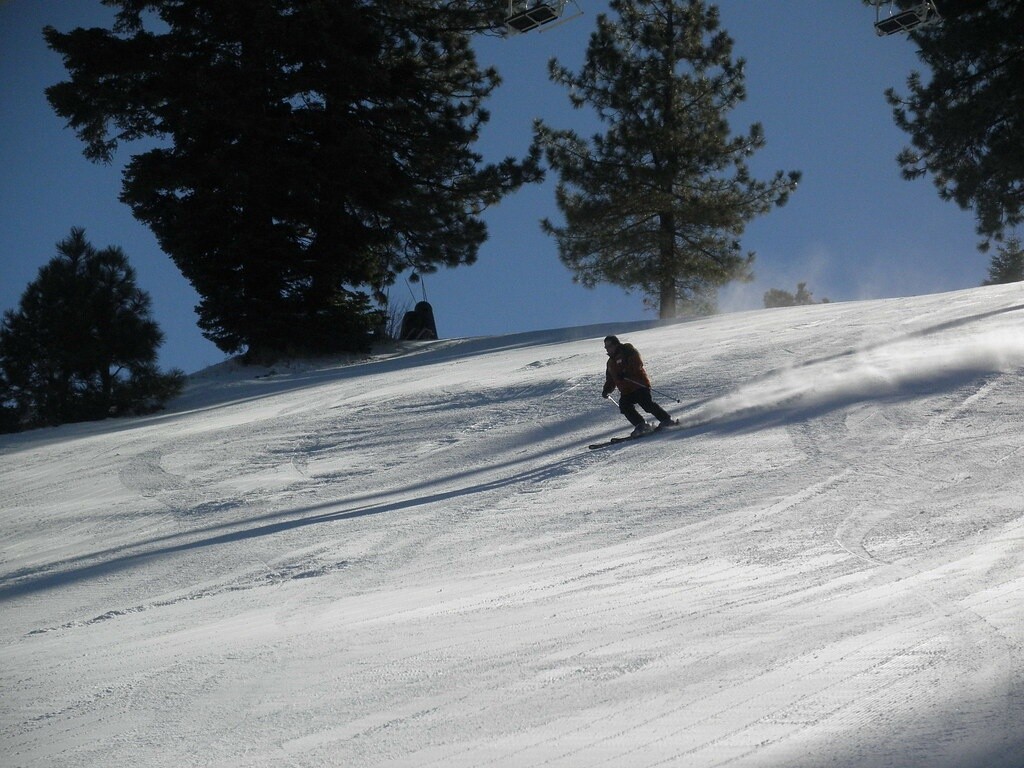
<box><xmin>585</xmin><ymin>417</ymin><xmax>684</xmax><ymax>454</ymax></box>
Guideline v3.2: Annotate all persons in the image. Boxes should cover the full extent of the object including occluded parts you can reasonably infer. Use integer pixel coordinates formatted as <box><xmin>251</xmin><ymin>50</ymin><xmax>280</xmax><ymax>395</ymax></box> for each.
<box><xmin>602</xmin><ymin>336</ymin><xmax>674</xmax><ymax>436</ymax></box>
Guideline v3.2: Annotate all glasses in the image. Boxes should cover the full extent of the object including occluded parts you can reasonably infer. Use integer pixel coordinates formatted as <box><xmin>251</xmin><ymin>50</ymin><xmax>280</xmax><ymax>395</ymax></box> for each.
<box><xmin>604</xmin><ymin>343</ymin><xmax>613</xmax><ymax>349</ymax></box>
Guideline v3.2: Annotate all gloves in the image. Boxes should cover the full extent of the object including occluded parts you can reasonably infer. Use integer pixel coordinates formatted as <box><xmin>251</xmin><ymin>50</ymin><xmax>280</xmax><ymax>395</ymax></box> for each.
<box><xmin>644</xmin><ymin>385</ymin><xmax>651</xmax><ymax>390</ymax></box>
<box><xmin>602</xmin><ymin>390</ymin><xmax>607</xmax><ymax>398</ymax></box>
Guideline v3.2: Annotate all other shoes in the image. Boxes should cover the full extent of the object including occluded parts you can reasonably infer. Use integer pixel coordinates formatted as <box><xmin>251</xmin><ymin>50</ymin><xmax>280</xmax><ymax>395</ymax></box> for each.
<box><xmin>655</xmin><ymin>420</ymin><xmax>675</xmax><ymax>430</ymax></box>
<box><xmin>631</xmin><ymin>423</ymin><xmax>651</xmax><ymax>437</ymax></box>
